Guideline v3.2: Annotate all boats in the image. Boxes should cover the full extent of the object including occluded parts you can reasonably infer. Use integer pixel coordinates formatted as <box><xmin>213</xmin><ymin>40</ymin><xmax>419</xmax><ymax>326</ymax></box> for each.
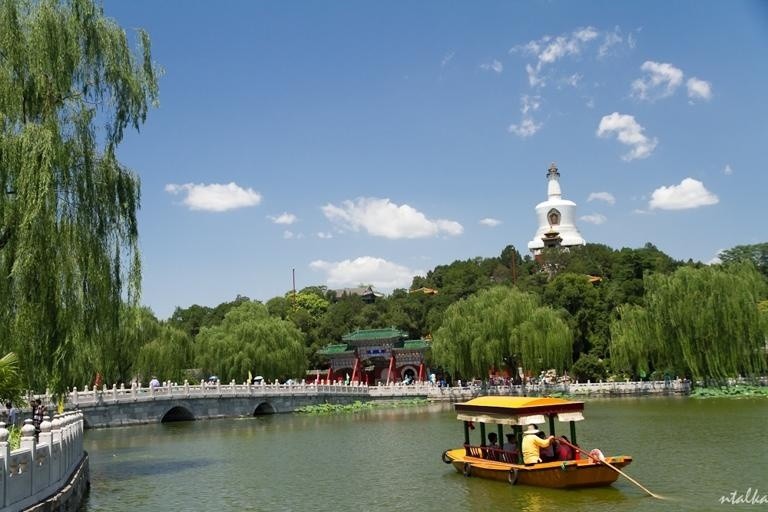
<box><xmin>441</xmin><ymin>391</ymin><xmax>635</xmax><ymax>488</ymax></box>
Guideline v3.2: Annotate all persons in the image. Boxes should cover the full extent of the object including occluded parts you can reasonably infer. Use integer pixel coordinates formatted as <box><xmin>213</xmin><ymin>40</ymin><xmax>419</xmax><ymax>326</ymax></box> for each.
<box><xmin>254</xmin><ymin>380</ymin><xmax>259</xmax><ymax>389</ymax></box>
<box><xmin>487</xmin><ymin>424</ymin><xmax>574</xmax><ymax>466</ymax></box>
<box><xmin>150</xmin><ymin>376</ymin><xmax>161</xmax><ymax>392</ymax></box>
<box><xmin>489</xmin><ymin>374</ymin><xmax>514</xmax><ymax>385</ymax></box>
<box><xmin>205</xmin><ymin>379</ymin><xmax>217</xmax><ymax>389</ymax></box>
<box><xmin>409</xmin><ymin>380</ymin><xmax>447</xmax><ymax>387</ymax></box>
<box><xmin>4</xmin><ymin>398</ymin><xmax>48</xmax><ymax>433</ymax></box>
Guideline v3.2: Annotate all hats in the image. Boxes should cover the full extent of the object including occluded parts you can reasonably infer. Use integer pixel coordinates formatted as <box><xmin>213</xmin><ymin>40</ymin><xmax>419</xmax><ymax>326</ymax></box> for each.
<box><xmin>523</xmin><ymin>425</ymin><xmax>539</xmax><ymax>434</ymax></box>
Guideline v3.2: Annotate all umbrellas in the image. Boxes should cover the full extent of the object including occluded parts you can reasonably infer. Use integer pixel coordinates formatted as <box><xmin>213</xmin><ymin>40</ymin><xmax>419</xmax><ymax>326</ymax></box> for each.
<box><xmin>254</xmin><ymin>375</ymin><xmax>263</xmax><ymax>380</ymax></box>
<box><xmin>209</xmin><ymin>376</ymin><xmax>219</xmax><ymax>381</ymax></box>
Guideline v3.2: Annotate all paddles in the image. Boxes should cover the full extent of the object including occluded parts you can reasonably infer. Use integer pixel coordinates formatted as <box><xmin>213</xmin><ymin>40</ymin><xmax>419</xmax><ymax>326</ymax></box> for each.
<box><xmin>554</xmin><ymin>435</ymin><xmax>674</xmax><ymax>501</ymax></box>
